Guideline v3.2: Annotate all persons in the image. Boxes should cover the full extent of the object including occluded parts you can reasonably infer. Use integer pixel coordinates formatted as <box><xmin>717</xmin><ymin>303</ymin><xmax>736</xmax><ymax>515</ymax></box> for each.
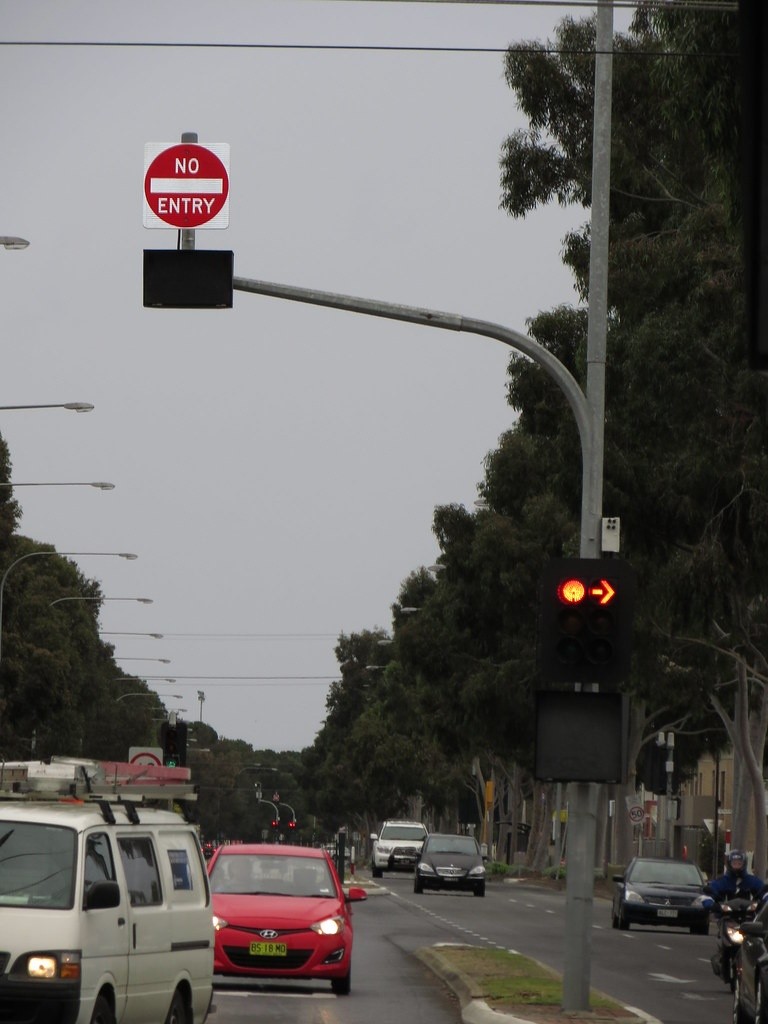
<box><xmin>700</xmin><ymin>849</ymin><xmax>766</xmax><ymax>962</ymax></box>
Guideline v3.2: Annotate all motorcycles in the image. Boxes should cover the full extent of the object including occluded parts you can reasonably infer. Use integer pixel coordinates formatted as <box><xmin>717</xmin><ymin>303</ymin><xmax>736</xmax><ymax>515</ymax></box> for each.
<box><xmin>711</xmin><ymin>897</ymin><xmax>761</xmax><ymax>993</ymax></box>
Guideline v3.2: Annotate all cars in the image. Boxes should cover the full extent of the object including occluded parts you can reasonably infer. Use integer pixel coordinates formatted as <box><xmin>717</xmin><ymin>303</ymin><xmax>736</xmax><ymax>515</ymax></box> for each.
<box><xmin>414</xmin><ymin>832</ymin><xmax>490</xmax><ymax>897</ymax></box>
<box><xmin>321</xmin><ymin>844</ymin><xmax>351</xmax><ymax>862</ymax></box>
<box><xmin>731</xmin><ymin>893</ymin><xmax>768</xmax><ymax>1024</ymax></box>
<box><xmin>204</xmin><ymin>842</ymin><xmax>368</xmax><ymax>996</ymax></box>
<box><xmin>611</xmin><ymin>856</ymin><xmax>710</xmax><ymax>936</ymax></box>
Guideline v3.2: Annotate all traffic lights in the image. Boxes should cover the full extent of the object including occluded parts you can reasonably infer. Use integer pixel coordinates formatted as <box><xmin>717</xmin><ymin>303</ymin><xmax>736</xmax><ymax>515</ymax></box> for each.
<box><xmin>165</xmin><ymin>754</ymin><xmax>181</xmax><ymax>768</ymax></box>
<box><xmin>544</xmin><ymin>558</ymin><xmax>631</xmax><ymax>701</ymax></box>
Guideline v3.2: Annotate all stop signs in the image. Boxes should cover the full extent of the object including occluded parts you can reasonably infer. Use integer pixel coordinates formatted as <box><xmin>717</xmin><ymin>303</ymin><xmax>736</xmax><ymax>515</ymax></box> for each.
<box><xmin>141</xmin><ymin>140</ymin><xmax>232</xmax><ymax>230</ymax></box>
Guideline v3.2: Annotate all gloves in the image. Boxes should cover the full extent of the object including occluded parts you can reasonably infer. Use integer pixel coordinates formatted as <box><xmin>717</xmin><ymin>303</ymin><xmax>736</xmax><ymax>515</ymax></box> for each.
<box><xmin>702</xmin><ymin>899</ymin><xmax>714</xmax><ymax>909</ymax></box>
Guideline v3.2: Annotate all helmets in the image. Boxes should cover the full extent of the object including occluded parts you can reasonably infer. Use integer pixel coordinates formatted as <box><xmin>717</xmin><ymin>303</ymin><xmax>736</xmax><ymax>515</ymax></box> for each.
<box><xmin>727</xmin><ymin>848</ymin><xmax>748</xmax><ymax>874</ymax></box>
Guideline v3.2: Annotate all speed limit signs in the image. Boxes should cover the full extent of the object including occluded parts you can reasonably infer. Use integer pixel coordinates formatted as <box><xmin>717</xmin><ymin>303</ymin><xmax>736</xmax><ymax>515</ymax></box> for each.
<box><xmin>628</xmin><ymin>804</ymin><xmax>649</xmax><ymax>828</ymax></box>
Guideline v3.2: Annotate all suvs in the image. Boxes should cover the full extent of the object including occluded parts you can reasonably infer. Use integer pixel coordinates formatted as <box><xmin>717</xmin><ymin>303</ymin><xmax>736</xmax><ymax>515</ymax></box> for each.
<box><xmin>368</xmin><ymin>818</ymin><xmax>428</xmax><ymax>878</ymax></box>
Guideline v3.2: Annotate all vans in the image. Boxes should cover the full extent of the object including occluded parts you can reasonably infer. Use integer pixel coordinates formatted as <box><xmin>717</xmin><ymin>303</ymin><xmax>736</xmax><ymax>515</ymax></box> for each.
<box><xmin>0</xmin><ymin>753</ymin><xmax>216</xmax><ymax>1024</ymax></box>
